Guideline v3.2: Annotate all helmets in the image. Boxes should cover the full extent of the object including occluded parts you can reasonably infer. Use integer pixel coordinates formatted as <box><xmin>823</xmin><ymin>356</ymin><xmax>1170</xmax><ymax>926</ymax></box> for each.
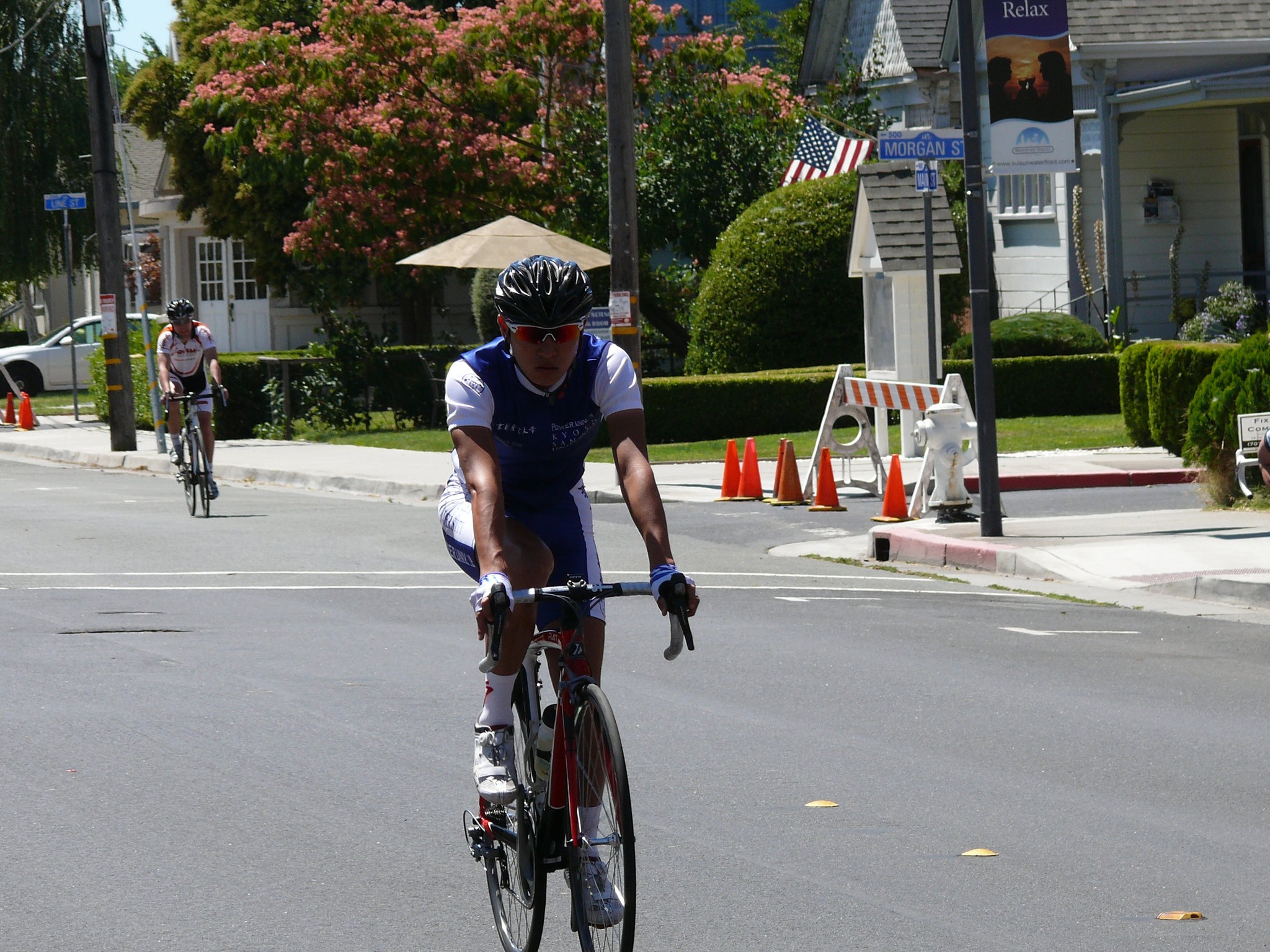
<box><xmin>494</xmin><ymin>254</ymin><xmax>592</xmax><ymax>327</ymax></box>
<box><xmin>165</xmin><ymin>298</ymin><xmax>194</xmax><ymax>320</ymax></box>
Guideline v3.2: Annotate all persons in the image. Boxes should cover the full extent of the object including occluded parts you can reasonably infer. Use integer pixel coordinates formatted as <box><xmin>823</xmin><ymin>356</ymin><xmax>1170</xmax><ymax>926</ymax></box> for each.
<box><xmin>438</xmin><ymin>256</ymin><xmax>700</xmax><ymax>929</ymax></box>
<box><xmin>156</xmin><ymin>298</ymin><xmax>229</xmax><ymax>499</ymax></box>
<box><xmin>988</xmin><ymin>51</ymin><xmax>1073</xmax><ymax>124</ymax></box>
<box><xmin>1257</xmin><ymin>429</ymin><xmax>1270</xmax><ymax>488</ymax></box>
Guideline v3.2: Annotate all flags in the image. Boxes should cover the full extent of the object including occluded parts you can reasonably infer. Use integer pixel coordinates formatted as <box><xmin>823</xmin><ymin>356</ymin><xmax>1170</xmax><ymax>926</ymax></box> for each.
<box><xmin>778</xmin><ymin>110</ymin><xmax>875</xmax><ymax>186</ymax></box>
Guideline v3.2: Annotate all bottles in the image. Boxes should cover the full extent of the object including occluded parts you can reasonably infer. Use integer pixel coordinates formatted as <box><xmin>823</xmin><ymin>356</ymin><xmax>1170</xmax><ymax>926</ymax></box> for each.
<box><xmin>534</xmin><ymin>703</ymin><xmax>557</xmax><ymax>780</ymax></box>
<box><xmin>186</xmin><ymin>432</ymin><xmax>192</xmax><ymax>447</ymax></box>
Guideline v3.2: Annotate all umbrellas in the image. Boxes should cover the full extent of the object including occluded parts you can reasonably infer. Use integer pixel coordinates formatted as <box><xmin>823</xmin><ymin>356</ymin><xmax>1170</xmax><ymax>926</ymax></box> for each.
<box><xmin>395</xmin><ymin>215</ymin><xmax>610</xmax><ymax>271</ymax></box>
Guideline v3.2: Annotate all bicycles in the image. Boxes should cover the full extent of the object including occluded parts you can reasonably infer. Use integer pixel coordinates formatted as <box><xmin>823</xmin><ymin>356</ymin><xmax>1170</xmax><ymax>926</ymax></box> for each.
<box><xmin>164</xmin><ymin>382</ymin><xmax>227</xmax><ymax>518</ymax></box>
<box><xmin>462</xmin><ymin>572</ymin><xmax>697</xmax><ymax>952</ymax></box>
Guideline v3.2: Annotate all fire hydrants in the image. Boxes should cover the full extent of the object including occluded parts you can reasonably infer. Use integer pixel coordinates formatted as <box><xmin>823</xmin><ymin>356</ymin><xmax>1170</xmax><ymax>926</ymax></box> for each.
<box><xmin>909</xmin><ymin>403</ymin><xmax>979</xmax><ymax>525</ymax></box>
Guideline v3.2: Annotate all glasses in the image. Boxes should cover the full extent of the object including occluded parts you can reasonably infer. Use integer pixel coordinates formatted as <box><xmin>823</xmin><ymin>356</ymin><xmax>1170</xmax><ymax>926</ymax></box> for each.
<box><xmin>169</xmin><ymin>317</ymin><xmax>194</xmax><ymax>324</ymax></box>
<box><xmin>496</xmin><ymin>310</ymin><xmax>586</xmax><ymax>344</ymax></box>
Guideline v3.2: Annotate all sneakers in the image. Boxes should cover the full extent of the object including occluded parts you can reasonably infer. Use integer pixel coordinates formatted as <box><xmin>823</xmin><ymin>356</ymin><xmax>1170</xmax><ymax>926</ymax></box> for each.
<box><xmin>474</xmin><ymin>710</ymin><xmax>518</xmax><ymax>805</ymax></box>
<box><xmin>207</xmin><ymin>481</ymin><xmax>218</xmax><ymax>498</ymax></box>
<box><xmin>170</xmin><ymin>445</ymin><xmax>183</xmax><ymax>464</ymax></box>
<box><xmin>579</xmin><ymin>857</ymin><xmax>624</xmax><ymax>927</ymax></box>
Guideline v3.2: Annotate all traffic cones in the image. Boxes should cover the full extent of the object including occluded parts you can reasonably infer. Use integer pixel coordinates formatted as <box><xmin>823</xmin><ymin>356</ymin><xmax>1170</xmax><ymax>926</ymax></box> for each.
<box><xmin>12</xmin><ymin>391</ymin><xmax>38</xmax><ymax>433</ymax></box>
<box><xmin>728</xmin><ymin>437</ymin><xmax>765</xmax><ymax>501</ymax></box>
<box><xmin>714</xmin><ymin>439</ymin><xmax>742</xmax><ymax>503</ymax></box>
<box><xmin>0</xmin><ymin>392</ymin><xmax>17</xmax><ymax>428</ymax></box>
<box><xmin>870</xmin><ymin>454</ymin><xmax>919</xmax><ymax>523</ymax></box>
<box><xmin>761</xmin><ymin>438</ymin><xmax>812</xmax><ymax>506</ymax></box>
<box><xmin>808</xmin><ymin>446</ymin><xmax>848</xmax><ymax>511</ymax></box>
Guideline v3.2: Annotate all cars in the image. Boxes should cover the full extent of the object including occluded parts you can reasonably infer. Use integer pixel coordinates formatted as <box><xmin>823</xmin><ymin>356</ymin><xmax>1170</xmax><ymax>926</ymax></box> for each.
<box><xmin>0</xmin><ymin>314</ymin><xmax>168</xmax><ymax>398</ymax></box>
